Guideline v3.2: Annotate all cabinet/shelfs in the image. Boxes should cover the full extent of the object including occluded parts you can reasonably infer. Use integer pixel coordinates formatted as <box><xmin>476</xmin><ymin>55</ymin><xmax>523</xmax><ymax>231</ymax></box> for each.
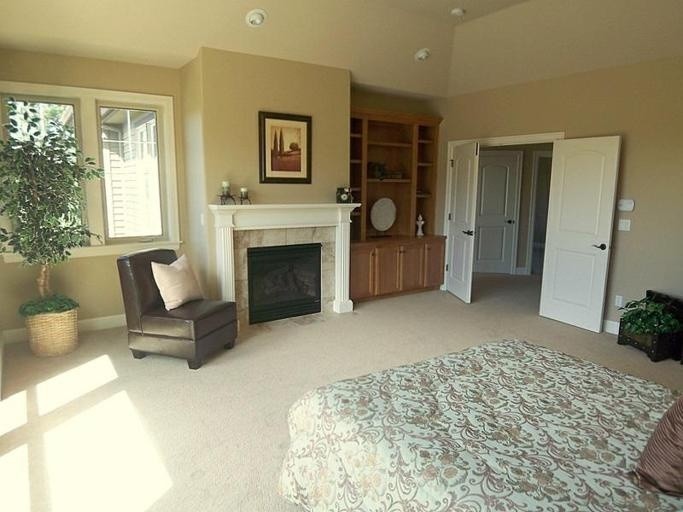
<box><xmin>351</xmin><ymin>105</ymin><xmax>447</xmax><ymax>301</ymax></box>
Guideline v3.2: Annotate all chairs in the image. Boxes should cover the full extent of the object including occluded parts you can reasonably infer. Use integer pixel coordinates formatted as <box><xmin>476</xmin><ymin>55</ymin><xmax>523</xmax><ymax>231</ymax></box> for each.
<box><xmin>119</xmin><ymin>249</ymin><xmax>238</xmax><ymax>369</ymax></box>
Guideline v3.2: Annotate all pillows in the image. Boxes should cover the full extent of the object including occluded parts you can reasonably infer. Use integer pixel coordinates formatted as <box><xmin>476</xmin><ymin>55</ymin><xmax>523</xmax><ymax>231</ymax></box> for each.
<box><xmin>145</xmin><ymin>253</ymin><xmax>208</xmax><ymax>311</ymax></box>
<box><xmin>628</xmin><ymin>394</ymin><xmax>681</xmax><ymax>500</ymax></box>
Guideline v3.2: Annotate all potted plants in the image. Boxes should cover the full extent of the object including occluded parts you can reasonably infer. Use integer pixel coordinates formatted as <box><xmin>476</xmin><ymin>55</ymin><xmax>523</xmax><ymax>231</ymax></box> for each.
<box><xmin>0</xmin><ymin>98</ymin><xmax>105</xmax><ymax>359</ymax></box>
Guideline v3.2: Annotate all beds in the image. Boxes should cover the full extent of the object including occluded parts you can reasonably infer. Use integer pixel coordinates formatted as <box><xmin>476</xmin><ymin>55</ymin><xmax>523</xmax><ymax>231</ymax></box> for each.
<box><xmin>307</xmin><ymin>340</ymin><xmax>683</xmax><ymax>512</ymax></box>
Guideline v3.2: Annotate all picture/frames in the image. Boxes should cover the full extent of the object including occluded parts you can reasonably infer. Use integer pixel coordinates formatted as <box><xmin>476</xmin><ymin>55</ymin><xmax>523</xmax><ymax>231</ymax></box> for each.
<box><xmin>257</xmin><ymin>110</ymin><xmax>312</xmax><ymax>183</ymax></box>
